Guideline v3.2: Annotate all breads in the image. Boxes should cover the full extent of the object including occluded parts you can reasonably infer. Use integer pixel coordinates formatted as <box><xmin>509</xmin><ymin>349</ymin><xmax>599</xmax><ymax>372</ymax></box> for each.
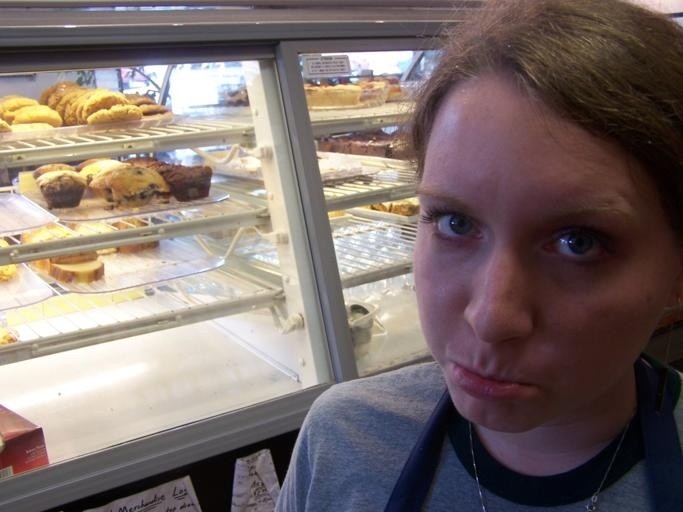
<box><xmin>229</xmin><ymin>77</ymin><xmax>421</xmax><ymax>217</ymax></box>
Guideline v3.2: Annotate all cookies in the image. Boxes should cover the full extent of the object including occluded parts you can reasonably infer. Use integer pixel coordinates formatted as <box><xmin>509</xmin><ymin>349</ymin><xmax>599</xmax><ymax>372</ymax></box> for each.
<box><xmin>0</xmin><ymin>82</ymin><xmax>212</xmax><ymax>134</ymax></box>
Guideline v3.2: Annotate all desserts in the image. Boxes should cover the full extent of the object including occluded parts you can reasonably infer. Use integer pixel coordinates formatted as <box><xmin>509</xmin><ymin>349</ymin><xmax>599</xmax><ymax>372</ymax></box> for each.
<box><xmin>31</xmin><ymin>156</ymin><xmax>212</xmax><ymax>210</ymax></box>
<box><xmin>1</xmin><ymin>218</ymin><xmax>160</xmax><ymax>282</ymax></box>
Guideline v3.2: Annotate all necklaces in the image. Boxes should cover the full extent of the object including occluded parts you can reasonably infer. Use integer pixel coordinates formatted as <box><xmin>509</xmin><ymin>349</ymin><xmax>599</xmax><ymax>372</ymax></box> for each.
<box><xmin>468</xmin><ymin>408</ymin><xmax>637</xmax><ymax>512</ymax></box>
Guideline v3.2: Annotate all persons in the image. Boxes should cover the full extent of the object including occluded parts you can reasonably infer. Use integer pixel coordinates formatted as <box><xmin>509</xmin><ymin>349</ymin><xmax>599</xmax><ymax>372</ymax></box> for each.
<box><xmin>274</xmin><ymin>0</ymin><xmax>683</xmax><ymax>512</ymax></box>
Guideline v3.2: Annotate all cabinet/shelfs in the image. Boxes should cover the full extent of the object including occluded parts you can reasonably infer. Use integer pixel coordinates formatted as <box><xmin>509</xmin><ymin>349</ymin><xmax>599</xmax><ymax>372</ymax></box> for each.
<box><xmin>0</xmin><ymin>7</ymin><xmax>461</xmax><ymax>512</ymax></box>
